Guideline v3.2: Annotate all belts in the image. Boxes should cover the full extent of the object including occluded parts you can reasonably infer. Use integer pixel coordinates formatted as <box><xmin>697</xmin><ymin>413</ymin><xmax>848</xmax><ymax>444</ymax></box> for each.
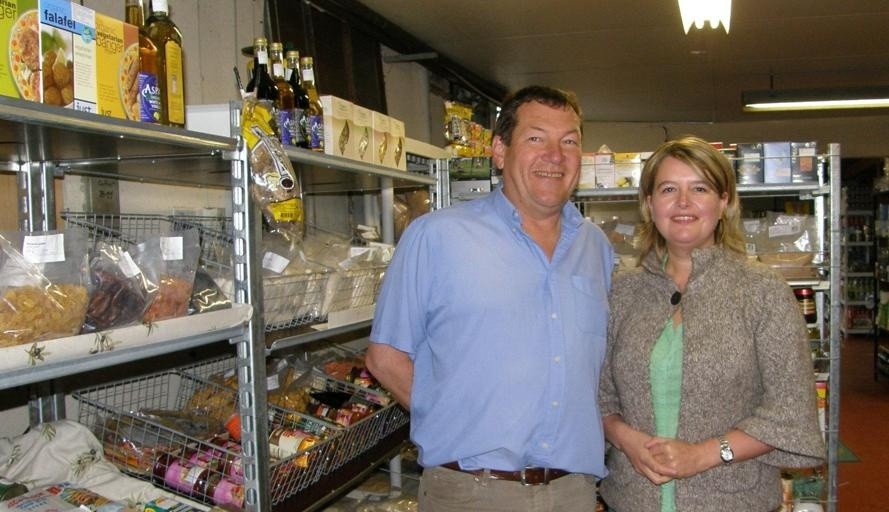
<box><xmin>440</xmin><ymin>462</ymin><xmax>572</xmax><ymax>488</ymax></box>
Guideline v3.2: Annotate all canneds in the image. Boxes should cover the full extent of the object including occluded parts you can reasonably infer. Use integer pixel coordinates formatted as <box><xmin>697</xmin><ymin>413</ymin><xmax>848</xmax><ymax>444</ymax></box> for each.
<box><xmin>151</xmin><ymin>403</ymin><xmax>374</xmax><ymax>510</ymax></box>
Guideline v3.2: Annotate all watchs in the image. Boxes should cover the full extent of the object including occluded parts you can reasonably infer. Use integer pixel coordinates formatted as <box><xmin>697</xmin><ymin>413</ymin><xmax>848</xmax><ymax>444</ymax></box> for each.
<box><xmin>715</xmin><ymin>432</ymin><xmax>736</xmax><ymax>469</ymax></box>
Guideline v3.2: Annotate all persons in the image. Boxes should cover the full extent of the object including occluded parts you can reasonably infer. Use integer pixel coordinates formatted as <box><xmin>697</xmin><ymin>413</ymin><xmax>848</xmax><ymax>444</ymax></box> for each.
<box><xmin>361</xmin><ymin>84</ymin><xmax>621</xmax><ymax>512</ymax></box>
<box><xmin>598</xmin><ymin>131</ymin><xmax>830</xmax><ymax>512</ymax></box>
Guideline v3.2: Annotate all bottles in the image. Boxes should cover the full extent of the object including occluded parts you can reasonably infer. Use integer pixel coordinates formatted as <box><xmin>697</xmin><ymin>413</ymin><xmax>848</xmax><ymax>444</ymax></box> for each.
<box><xmin>124</xmin><ymin>1</ymin><xmax>188</xmax><ymax>130</ymax></box>
<box><xmin>875</xmin><ymin>343</ymin><xmax>889</xmax><ymax>378</ymax></box>
<box><xmin>833</xmin><ymin>215</ymin><xmax>874</xmax><ymax>330</ymax></box>
<box><xmin>246</xmin><ymin>34</ymin><xmax>325</xmax><ymax>152</ymax></box>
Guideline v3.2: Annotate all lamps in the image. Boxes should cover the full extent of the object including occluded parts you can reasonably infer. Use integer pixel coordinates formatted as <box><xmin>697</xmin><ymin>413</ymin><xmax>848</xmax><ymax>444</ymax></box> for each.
<box><xmin>740</xmin><ymin>74</ymin><xmax>889</xmax><ymax>112</ymax></box>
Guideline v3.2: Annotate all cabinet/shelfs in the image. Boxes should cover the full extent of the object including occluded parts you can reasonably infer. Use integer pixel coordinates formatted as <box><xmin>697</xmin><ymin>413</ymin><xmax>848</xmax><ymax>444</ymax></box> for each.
<box><xmin>841</xmin><ymin>206</ymin><xmax>875</xmax><ymax>340</ymax></box>
<box><xmin>872</xmin><ymin>184</ymin><xmax>889</xmax><ymax>384</ymax></box>
